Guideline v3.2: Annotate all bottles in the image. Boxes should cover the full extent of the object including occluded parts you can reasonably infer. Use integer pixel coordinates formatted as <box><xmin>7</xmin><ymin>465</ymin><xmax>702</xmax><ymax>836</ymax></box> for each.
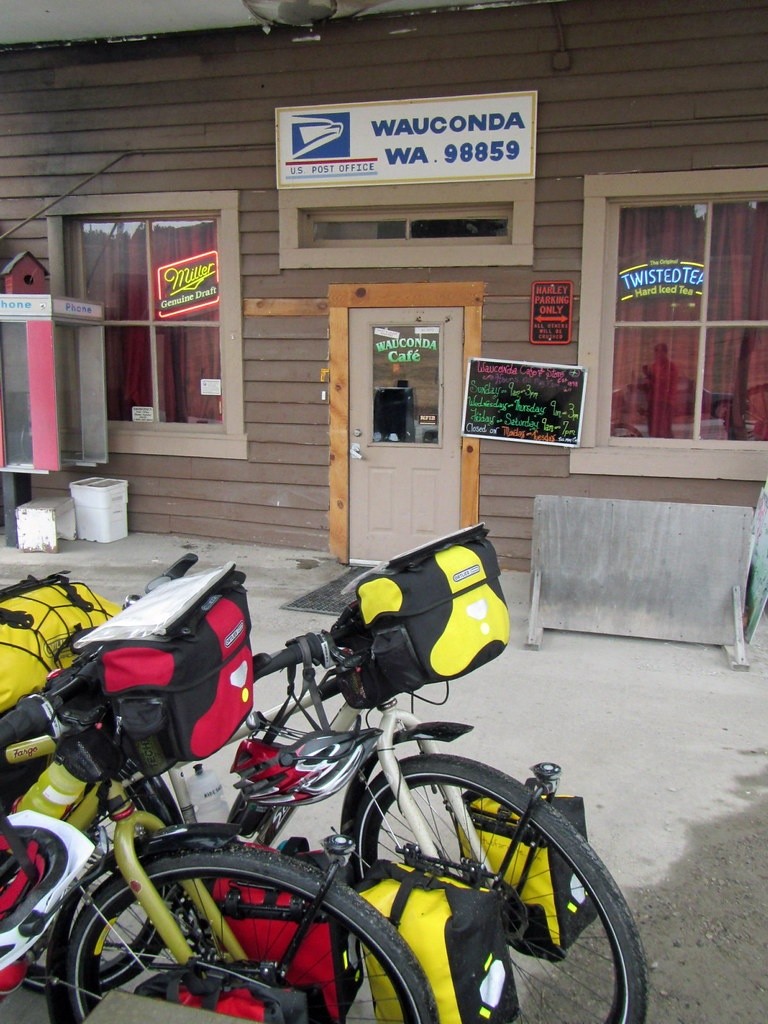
<box><xmin>185</xmin><ymin>764</ymin><xmax>231</xmax><ymax>825</ymax></box>
<box><xmin>11</xmin><ymin>756</ymin><xmax>96</xmax><ymax>825</ymax></box>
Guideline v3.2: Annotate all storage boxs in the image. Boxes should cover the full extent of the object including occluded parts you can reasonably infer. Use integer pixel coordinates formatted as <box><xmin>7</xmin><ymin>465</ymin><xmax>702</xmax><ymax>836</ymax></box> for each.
<box><xmin>74</xmin><ymin>506</ymin><xmax>128</xmax><ymax>543</ymax></box>
<box><xmin>68</xmin><ymin>477</ymin><xmax>129</xmax><ymax>508</ymax></box>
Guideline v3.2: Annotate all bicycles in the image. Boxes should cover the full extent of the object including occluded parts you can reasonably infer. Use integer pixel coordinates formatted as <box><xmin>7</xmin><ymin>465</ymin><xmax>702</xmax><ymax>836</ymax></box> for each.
<box><xmin>0</xmin><ymin>548</ymin><xmax>653</xmax><ymax>1024</ymax></box>
<box><xmin>723</xmin><ymin>384</ymin><xmax>768</xmax><ymax>441</ymax></box>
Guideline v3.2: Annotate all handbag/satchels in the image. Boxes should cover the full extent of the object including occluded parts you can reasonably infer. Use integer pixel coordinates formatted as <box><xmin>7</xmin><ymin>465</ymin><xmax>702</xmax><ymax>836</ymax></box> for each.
<box><xmin>0</xmin><ymin>569</ymin><xmax>126</xmax><ymax>718</ymax></box>
<box><xmin>355</xmin><ymin>859</ymin><xmax>519</xmax><ymax>1024</ymax></box>
<box><xmin>209</xmin><ymin>835</ymin><xmax>364</xmax><ymax>1023</ymax></box>
<box><xmin>453</xmin><ymin>789</ymin><xmax>598</xmax><ymax>949</ymax></box>
<box><xmin>133</xmin><ymin>955</ymin><xmax>309</xmax><ymax>1024</ymax></box>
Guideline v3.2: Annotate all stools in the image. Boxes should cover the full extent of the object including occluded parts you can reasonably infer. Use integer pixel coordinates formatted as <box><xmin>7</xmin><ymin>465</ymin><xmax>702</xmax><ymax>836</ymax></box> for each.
<box><xmin>15</xmin><ymin>496</ymin><xmax>77</xmax><ymax>552</ymax></box>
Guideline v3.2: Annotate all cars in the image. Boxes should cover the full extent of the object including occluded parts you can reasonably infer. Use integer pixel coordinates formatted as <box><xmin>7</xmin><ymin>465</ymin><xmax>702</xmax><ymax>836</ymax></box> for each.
<box><xmin>615</xmin><ymin>379</ymin><xmax>736</xmax><ymax>419</ymax></box>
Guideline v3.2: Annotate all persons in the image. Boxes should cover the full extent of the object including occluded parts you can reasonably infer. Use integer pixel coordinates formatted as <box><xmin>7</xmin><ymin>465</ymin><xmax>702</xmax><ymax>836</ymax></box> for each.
<box><xmin>642</xmin><ymin>343</ymin><xmax>677</xmax><ymax>437</ymax></box>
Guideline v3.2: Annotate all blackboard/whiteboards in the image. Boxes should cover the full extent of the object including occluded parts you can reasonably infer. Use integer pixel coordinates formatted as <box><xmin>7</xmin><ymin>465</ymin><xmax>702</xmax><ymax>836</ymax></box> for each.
<box><xmin>461</xmin><ymin>357</ymin><xmax>588</xmax><ymax>449</ymax></box>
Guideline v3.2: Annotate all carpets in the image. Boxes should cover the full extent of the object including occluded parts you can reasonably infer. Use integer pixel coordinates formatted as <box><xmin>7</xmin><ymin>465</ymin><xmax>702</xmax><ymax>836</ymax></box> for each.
<box><xmin>280</xmin><ymin>567</ymin><xmax>376</xmax><ymax>615</ymax></box>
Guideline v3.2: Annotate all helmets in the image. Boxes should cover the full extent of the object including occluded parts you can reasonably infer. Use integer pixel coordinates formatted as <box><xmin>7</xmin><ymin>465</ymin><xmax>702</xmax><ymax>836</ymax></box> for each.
<box><xmin>0</xmin><ymin>810</ymin><xmax>95</xmax><ymax>996</ymax></box>
<box><xmin>229</xmin><ymin>710</ymin><xmax>384</xmax><ymax>807</ymax></box>
